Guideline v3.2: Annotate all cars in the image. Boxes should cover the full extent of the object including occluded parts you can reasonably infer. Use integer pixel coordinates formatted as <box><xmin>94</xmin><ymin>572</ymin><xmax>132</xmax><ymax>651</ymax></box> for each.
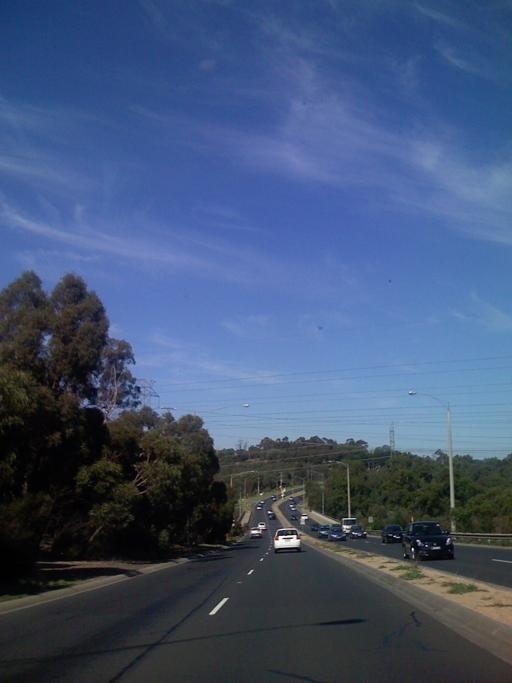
<box><xmin>401</xmin><ymin>520</ymin><xmax>455</xmax><ymax>561</ymax></box>
<box><xmin>380</xmin><ymin>523</ymin><xmax>403</xmax><ymax>544</ymax></box>
<box><xmin>248</xmin><ymin>493</ymin><xmax>368</xmax><ymax>541</ymax></box>
<box><xmin>272</xmin><ymin>527</ymin><xmax>302</xmax><ymax>553</ymax></box>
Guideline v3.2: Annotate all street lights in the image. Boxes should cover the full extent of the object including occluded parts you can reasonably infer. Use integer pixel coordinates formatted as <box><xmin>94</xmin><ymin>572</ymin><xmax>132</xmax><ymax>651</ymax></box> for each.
<box><xmin>327</xmin><ymin>459</ymin><xmax>351</xmax><ymax>519</ymax></box>
<box><xmin>230</xmin><ymin>466</ymin><xmax>325</xmax><ymax>526</ymax></box>
<box><xmin>408</xmin><ymin>389</ymin><xmax>458</xmax><ymax>534</ymax></box>
<box><xmin>194</xmin><ymin>403</ymin><xmax>250</xmax><ymax>416</ymax></box>
